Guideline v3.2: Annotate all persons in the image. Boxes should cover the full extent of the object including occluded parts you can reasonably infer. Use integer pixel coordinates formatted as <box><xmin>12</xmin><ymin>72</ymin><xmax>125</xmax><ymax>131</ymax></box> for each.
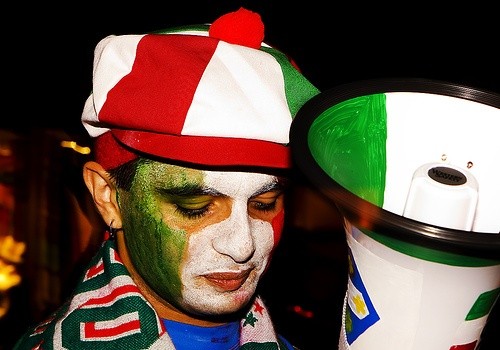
<box><xmin>9</xmin><ymin>6</ymin><xmax>319</xmax><ymax>349</ymax></box>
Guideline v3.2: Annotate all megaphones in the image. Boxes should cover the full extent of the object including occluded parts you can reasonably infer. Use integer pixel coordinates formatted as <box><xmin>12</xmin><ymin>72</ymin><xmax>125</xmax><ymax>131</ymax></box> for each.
<box><xmin>290</xmin><ymin>79</ymin><xmax>500</xmax><ymax>350</ymax></box>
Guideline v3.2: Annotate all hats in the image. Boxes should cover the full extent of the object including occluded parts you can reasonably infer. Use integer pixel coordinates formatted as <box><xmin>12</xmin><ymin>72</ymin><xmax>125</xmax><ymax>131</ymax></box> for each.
<box><xmin>81</xmin><ymin>7</ymin><xmax>320</xmax><ymax>171</ymax></box>
